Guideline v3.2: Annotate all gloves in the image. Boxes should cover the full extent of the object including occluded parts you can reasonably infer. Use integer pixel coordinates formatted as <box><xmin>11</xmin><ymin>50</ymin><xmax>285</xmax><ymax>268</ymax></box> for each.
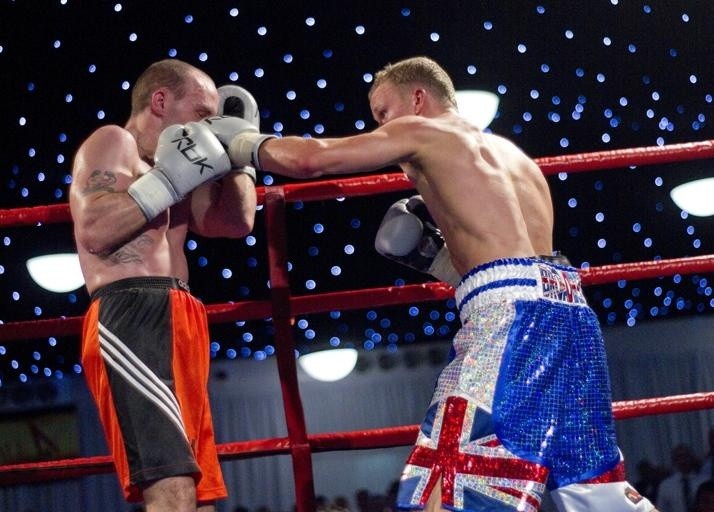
<box><xmin>128</xmin><ymin>121</ymin><xmax>234</xmax><ymax>225</ymax></box>
<box><xmin>373</xmin><ymin>191</ymin><xmax>463</xmax><ymax>288</ymax></box>
<box><xmin>196</xmin><ymin>81</ymin><xmax>278</xmax><ymax>183</ymax></box>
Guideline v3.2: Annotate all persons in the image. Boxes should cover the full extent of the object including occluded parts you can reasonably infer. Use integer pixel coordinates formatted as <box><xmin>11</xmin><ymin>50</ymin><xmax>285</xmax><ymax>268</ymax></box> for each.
<box><xmin>614</xmin><ymin>430</ymin><xmax>714</xmax><ymax>512</ymax></box>
<box><xmin>315</xmin><ymin>479</ymin><xmax>400</xmax><ymax>512</ymax></box>
<box><xmin>65</xmin><ymin>58</ymin><xmax>261</xmax><ymax>512</ymax></box>
<box><xmin>195</xmin><ymin>56</ymin><xmax>662</xmax><ymax>511</ymax></box>
